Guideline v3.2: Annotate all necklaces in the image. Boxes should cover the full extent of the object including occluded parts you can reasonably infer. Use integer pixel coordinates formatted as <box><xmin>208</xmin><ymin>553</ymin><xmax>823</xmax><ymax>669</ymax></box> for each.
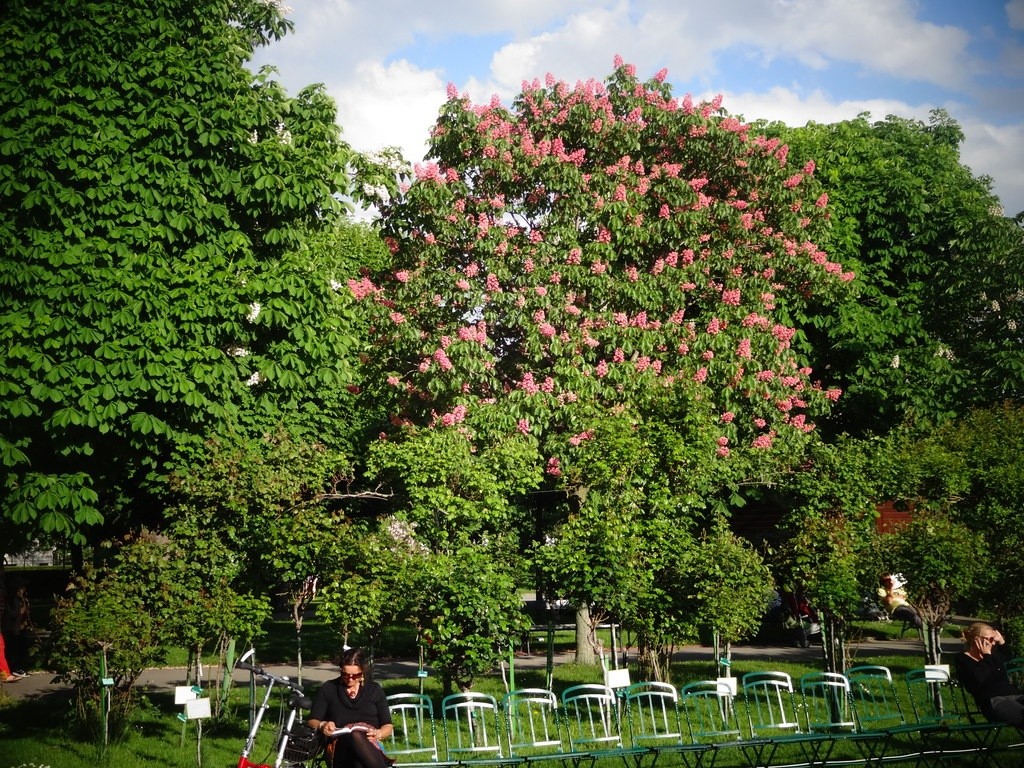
<box><xmin>348</xmin><ymin>687</ymin><xmax>357</xmax><ymax>696</ymax></box>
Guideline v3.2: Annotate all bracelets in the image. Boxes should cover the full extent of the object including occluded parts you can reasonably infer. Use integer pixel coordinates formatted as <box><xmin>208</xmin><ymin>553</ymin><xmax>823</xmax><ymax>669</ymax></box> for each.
<box><xmin>319</xmin><ymin>721</ymin><xmax>326</xmax><ymax>729</ymax></box>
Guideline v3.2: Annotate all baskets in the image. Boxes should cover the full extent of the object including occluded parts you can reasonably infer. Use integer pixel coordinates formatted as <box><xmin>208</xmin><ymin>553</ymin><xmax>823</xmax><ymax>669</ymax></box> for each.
<box><xmin>276</xmin><ymin>717</ymin><xmax>327</xmax><ymax>763</ymax></box>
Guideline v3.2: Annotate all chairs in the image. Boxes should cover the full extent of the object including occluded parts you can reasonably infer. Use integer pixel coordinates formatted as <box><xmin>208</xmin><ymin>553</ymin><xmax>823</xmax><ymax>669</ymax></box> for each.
<box><xmin>957</xmin><ymin>670</ymin><xmax>1024</xmax><ymax>768</ymax></box>
<box><xmin>906</xmin><ymin>669</ymin><xmax>1008</xmax><ymax>768</ymax></box>
<box><xmin>384</xmin><ymin>693</ymin><xmax>459</xmax><ymax>768</ymax></box>
<box><xmin>624</xmin><ymin>680</ymin><xmax>712</xmax><ymax>768</ymax></box>
<box><xmin>681</xmin><ymin>681</ymin><xmax>772</xmax><ymax>768</ymax></box>
<box><xmin>743</xmin><ymin>671</ymin><xmax>830</xmax><ymax>768</ymax></box>
<box><xmin>311</xmin><ymin>731</ymin><xmax>328</xmax><ymax>768</ymax></box>
<box><xmin>845</xmin><ymin>665</ymin><xmax>940</xmax><ymax>768</ymax></box>
<box><xmin>562</xmin><ymin>684</ymin><xmax>650</xmax><ymax>768</ymax></box>
<box><xmin>501</xmin><ymin>688</ymin><xmax>589</xmax><ymax>768</ymax></box>
<box><xmin>442</xmin><ymin>692</ymin><xmax>525</xmax><ymax>768</ymax></box>
<box><xmin>800</xmin><ymin>672</ymin><xmax>891</xmax><ymax>768</ymax></box>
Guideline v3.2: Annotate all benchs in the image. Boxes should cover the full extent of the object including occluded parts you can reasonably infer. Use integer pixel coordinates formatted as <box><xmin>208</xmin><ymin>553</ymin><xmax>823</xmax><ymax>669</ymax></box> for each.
<box><xmin>844</xmin><ymin>612</ymin><xmax>922</xmax><ymax>641</ymax></box>
<box><xmin>511</xmin><ymin>601</ymin><xmax>623</xmax><ymax>653</ymax></box>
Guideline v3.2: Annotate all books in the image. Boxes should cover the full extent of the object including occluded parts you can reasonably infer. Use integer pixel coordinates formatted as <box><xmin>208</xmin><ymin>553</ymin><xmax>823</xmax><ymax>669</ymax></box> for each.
<box><xmin>331</xmin><ymin>726</ymin><xmax>369</xmax><ymax>736</ymax></box>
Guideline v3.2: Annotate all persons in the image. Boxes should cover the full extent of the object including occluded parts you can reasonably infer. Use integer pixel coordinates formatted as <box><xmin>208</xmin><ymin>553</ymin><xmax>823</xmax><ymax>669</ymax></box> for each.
<box><xmin>307</xmin><ymin>649</ymin><xmax>396</xmax><ymax>768</ymax></box>
<box><xmin>0</xmin><ymin>633</ymin><xmax>22</xmax><ymax>683</ymax></box>
<box><xmin>955</xmin><ymin>622</ymin><xmax>1024</xmax><ymax>740</ymax></box>
<box><xmin>879</xmin><ymin>573</ymin><xmax>923</xmax><ymax>629</ymax></box>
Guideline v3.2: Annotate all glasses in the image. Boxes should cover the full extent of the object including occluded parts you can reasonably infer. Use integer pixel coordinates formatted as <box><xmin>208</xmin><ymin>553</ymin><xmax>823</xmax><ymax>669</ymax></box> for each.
<box><xmin>978</xmin><ymin>635</ymin><xmax>994</xmax><ymax>644</ymax></box>
<box><xmin>340</xmin><ymin>671</ymin><xmax>362</xmax><ymax>680</ymax></box>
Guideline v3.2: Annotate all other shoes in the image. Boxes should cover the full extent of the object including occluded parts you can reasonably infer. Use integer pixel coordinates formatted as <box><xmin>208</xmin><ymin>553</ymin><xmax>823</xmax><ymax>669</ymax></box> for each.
<box><xmin>2</xmin><ymin>675</ymin><xmax>22</xmax><ymax>683</ymax></box>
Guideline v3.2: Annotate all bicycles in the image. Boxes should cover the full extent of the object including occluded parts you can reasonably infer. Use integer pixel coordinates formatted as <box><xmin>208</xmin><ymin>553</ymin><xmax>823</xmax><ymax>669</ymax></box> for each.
<box><xmin>235</xmin><ymin>662</ymin><xmax>327</xmax><ymax>768</ymax></box>
